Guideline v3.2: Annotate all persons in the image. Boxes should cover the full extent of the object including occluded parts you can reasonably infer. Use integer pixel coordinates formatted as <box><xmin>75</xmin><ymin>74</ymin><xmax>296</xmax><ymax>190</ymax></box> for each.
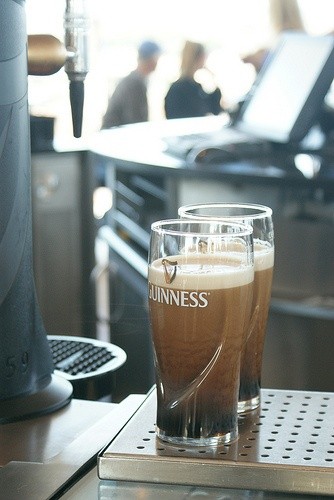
<box><xmin>99</xmin><ymin>42</ymin><xmax>160</xmax><ymax>131</ymax></box>
<box><xmin>165</xmin><ymin>41</ymin><xmax>224</xmax><ymax>119</ymax></box>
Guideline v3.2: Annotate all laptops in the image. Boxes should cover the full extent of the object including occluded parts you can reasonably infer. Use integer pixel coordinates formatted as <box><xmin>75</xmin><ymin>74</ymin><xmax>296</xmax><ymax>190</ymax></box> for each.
<box><xmin>158</xmin><ymin>31</ymin><xmax>334</xmax><ymax>163</ymax></box>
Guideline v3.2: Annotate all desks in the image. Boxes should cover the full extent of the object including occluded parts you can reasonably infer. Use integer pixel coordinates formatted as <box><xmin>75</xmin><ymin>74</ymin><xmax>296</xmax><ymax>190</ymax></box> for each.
<box><xmin>89</xmin><ymin>114</ymin><xmax>334</xmax><ymax>392</ymax></box>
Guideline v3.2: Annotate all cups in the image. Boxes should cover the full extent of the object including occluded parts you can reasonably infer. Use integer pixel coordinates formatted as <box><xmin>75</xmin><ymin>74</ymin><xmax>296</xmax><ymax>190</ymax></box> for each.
<box><xmin>178</xmin><ymin>203</ymin><xmax>274</xmax><ymax>412</ymax></box>
<box><xmin>148</xmin><ymin>219</ymin><xmax>254</xmax><ymax>446</ymax></box>
